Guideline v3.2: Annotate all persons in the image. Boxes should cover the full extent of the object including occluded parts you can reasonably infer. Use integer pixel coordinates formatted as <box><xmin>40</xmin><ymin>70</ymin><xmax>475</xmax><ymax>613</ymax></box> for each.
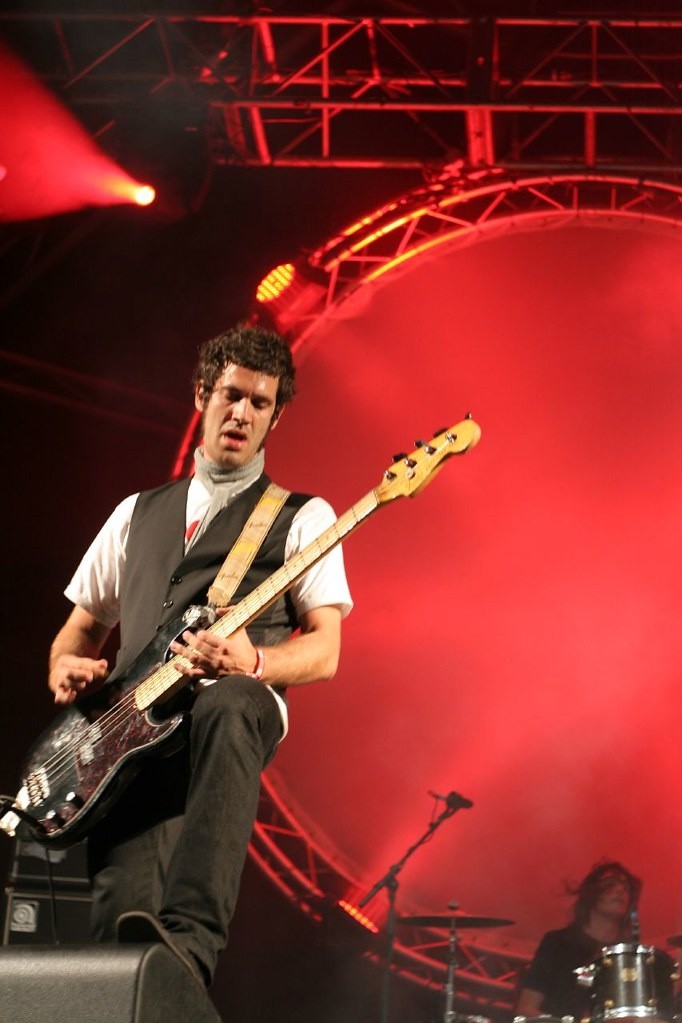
<box><xmin>49</xmin><ymin>328</ymin><xmax>353</xmax><ymax>984</ymax></box>
<box><xmin>517</xmin><ymin>862</ymin><xmax>643</xmax><ymax>1018</ymax></box>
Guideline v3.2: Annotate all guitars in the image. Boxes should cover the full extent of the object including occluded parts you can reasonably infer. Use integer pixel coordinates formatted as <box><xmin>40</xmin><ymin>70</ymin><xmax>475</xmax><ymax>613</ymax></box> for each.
<box><xmin>17</xmin><ymin>412</ymin><xmax>484</xmax><ymax>852</ymax></box>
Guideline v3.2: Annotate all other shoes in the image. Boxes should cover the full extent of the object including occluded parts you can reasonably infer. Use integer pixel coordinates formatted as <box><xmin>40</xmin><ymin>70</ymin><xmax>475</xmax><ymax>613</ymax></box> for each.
<box><xmin>116</xmin><ymin>912</ymin><xmax>205</xmax><ymax>989</ymax></box>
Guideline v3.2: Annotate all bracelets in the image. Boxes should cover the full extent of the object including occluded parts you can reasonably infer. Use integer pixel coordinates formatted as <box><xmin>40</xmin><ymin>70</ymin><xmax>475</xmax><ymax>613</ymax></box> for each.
<box><xmin>253</xmin><ymin>648</ymin><xmax>265</xmax><ymax>681</ymax></box>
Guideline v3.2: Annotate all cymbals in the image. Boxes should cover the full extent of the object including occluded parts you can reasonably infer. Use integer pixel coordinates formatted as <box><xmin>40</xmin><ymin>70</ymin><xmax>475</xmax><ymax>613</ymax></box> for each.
<box><xmin>391</xmin><ymin>907</ymin><xmax>518</xmax><ymax>929</ymax></box>
<box><xmin>665</xmin><ymin>934</ymin><xmax>682</xmax><ymax>947</ymax></box>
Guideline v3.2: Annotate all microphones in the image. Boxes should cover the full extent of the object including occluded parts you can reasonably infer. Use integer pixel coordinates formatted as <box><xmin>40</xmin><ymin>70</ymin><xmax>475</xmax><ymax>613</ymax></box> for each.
<box><xmin>434</xmin><ymin>792</ymin><xmax>473</xmax><ymax>809</ymax></box>
<box><xmin>631</xmin><ymin>908</ymin><xmax>640</xmax><ymax>943</ymax></box>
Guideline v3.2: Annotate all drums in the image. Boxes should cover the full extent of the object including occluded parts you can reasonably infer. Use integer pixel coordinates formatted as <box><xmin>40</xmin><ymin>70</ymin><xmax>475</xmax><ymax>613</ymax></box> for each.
<box><xmin>586</xmin><ymin>942</ymin><xmax>677</xmax><ymax>1023</ymax></box>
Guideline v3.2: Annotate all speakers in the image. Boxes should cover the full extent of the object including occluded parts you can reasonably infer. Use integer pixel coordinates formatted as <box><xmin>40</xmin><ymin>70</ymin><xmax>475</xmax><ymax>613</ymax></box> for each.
<box><xmin>0</xmin><ymin>887</ymin><xmax>95</xmax><ymax>951</ymax></box>
<box><xmin>0</xmin><ymin>944</ymin><xmax>221</xmax><ymax>1023</ymax></box>
